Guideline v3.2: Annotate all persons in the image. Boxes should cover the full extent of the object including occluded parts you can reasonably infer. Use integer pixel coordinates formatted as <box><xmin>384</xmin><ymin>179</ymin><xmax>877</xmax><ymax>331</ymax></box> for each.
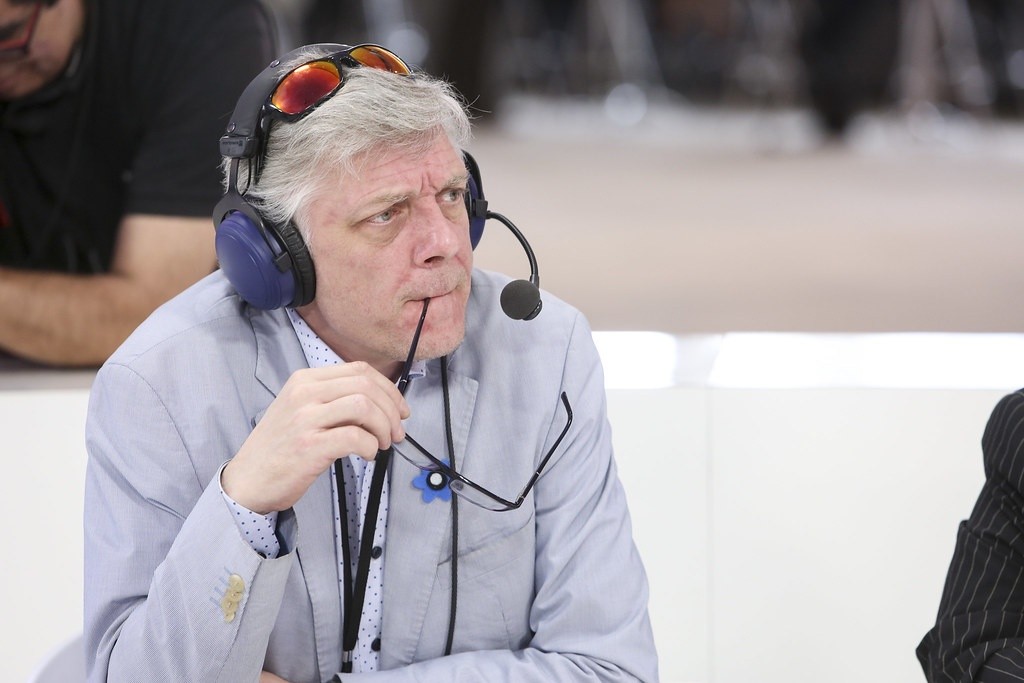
<box><xmin>81</xmin><ymin>43</ymin><xmax>658</xmax><ymax>683</ymax></box>
<box><xmin>914</xmin><ymin>389</ymin><xmax>1024</xmax><ymax>683</ymax></box>
<box><xmin>0</xmin><ymin>0</ymin><xmax>281</xmax><ymax>369</ymax></box>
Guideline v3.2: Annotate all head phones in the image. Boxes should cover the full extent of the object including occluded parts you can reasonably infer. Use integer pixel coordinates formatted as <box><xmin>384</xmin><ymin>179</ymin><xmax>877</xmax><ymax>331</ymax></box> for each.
<box><xmin>213</xmin><ymin>43</ymin><xmax>488</xmax><ymax>311</ymax></box>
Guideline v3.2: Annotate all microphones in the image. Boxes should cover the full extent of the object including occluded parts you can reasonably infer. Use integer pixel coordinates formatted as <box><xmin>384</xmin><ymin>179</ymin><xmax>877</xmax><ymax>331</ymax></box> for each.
<box><xmin>485</xmin><ymin>207</ymin><xmax>542</xmax><ymax>321</ymax></box>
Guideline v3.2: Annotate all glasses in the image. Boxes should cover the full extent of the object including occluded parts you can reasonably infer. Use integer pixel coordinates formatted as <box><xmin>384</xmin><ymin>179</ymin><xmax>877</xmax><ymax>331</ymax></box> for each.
<box><xmin>389</xmin><ymin>297</ymin><xmax>572</xmax><ymax>512</ymax></box>
<box><xmin>0</xmin><ymin>0</ymin><xmax>42</xmax><ymax>64</ymax></box>
<box><xmin>256</xmin><ymin>43</ymin><xmax>413</xmax><ymax>131</ymax></box>
